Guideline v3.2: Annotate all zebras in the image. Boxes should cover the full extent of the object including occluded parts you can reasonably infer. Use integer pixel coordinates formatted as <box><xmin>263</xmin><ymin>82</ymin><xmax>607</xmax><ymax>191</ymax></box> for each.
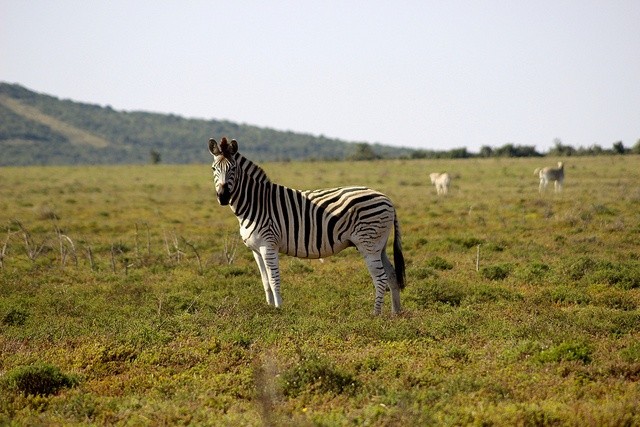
<box><xmin>208</xmin><ymin>137</ymin><xmax>406</xmax><ymax>316</ymax></box>
